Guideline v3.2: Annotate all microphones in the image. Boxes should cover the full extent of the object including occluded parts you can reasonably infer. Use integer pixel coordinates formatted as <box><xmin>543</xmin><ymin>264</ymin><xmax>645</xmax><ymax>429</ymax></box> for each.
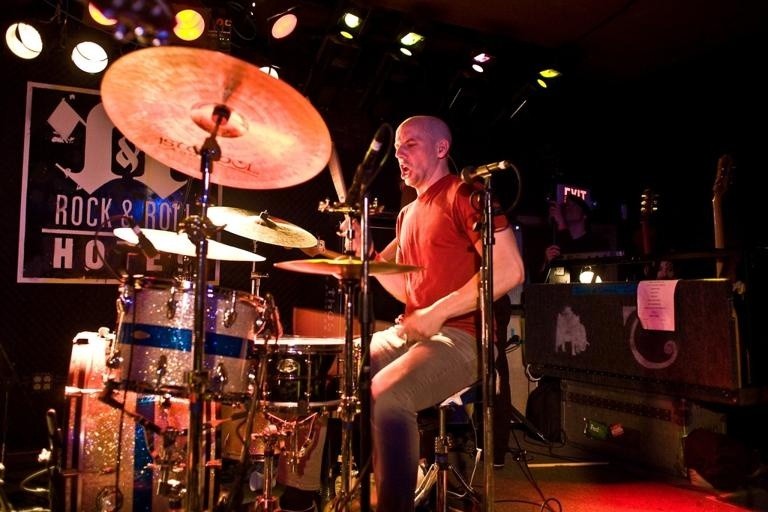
<box><xmin>346</xmin><ymin>123</ymin><xmax>387</xmax><ymax>207</ymax></box>
<box><xmin>130</xmin><ymin>220</ymin><xmax>158</xmax><ymax>258</ymax></box>
<box><xmin>265</xmin><ymin>293</ymin><xmax>283</xmax><ymax>339</ymax></box>
<box><xmin>460</xmin><ymin>159</ymin><xmax>509</xmax><ymax>185</ymax></box>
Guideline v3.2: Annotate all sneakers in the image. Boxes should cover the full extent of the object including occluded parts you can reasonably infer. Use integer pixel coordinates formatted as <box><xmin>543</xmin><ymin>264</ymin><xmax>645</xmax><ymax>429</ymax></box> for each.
<box><xmin>468</xmin><ymin>441</ymin><xmax>506</xmax><ymax>468</ymax></box>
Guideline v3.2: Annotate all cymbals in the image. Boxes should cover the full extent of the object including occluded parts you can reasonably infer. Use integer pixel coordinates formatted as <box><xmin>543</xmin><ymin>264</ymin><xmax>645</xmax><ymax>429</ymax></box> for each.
<box><xmin>273</xmin><ymin>259</ymin><xmax>422</xmax><ymax>276</ymax></box>
<box><xmin>100</xmin><ymin>47</ymin><xmax>332</xmax><ymax>191</ymax></box>
<box><xmin>113</xmin><ymin>227</ymin><xmax>267</xmax><ymax>263</ymax></box>
<box><xmin>207</xmin><ymin>206</ymin><xmax>318</xmax><ymax>249</ymax></box>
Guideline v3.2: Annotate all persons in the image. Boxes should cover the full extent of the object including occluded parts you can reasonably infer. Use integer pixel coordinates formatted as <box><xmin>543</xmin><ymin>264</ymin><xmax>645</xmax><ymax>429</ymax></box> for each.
<box><xmin>533</xmin><ymin>192</ymin><xmax>592</xmax><ymax>282</ymax></box>
<box><xmin>335</xmin><ymin>115</ymin><xmax>527</xmax><ymax>511</ymax></box>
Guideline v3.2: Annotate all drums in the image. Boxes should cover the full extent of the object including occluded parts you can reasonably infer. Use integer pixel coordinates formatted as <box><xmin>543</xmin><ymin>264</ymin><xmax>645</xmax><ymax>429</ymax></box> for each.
<box><xmin>276</xmin><ymin>401</ymin><xmax>353</xmax><ymax>491</ymax></box>
<box><xmin>49</xmin><ymin>330</ymin><xmax>222</xmax><ymax>511</ymax></box>
<box><xmin>252</xmin><ymin>336</ymin><xmax>362</xmax><ymax>409</ymax></box>
<box><xmin>222</xmin><ymin>402</ymin><xmax>283</xmax><ymax>465</ymax></box>
<box><xmin>102</xmin><ymin>273</ymin><xmax>270</xmax><ymax>408</ymax></box>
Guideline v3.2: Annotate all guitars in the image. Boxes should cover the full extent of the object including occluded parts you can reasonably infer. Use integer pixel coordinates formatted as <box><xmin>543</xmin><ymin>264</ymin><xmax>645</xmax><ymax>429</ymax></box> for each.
<box><xmin>639</xmin><ymin>183</ymin><xmax>657</xmax><ymax>280</ymax></box>
<box><xmin>712</xmin><ymin>152</ymin><xmax>730</xmax><ymax>278</ymax></box>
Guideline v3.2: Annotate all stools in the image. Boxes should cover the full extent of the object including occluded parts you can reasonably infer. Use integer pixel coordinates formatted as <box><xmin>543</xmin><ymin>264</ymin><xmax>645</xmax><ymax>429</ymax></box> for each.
<box><xmin>411</xmin><ymin>379</ymin><xmax>488</xmax><ymax>512</ymax></box>
